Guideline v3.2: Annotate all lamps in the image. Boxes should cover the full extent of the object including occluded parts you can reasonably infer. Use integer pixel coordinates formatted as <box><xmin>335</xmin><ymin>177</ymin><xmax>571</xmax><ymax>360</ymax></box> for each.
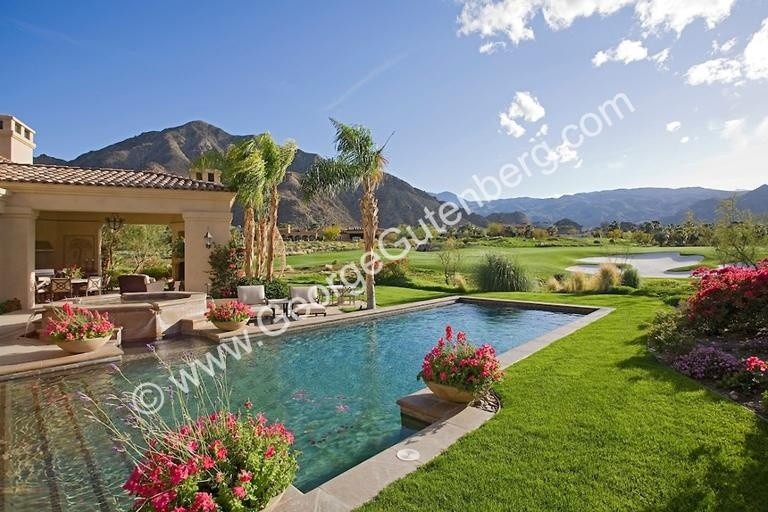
<box><xmin>104</xmin><ymin>213</ymin><xmax>124</xmax><ymax>231</ymax></box>
<box><xmin>204</xmin><ymin>232</ymin><xmax>212</xmax><ymax>249</ymax></box>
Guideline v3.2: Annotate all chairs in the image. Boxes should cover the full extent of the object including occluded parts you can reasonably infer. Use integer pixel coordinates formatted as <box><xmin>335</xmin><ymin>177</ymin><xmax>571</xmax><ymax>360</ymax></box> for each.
<box><xmin>34</xmin><ymin>271</ymin><xmax>111</xmax><ymax>301</ymax></box>
<box><xmin>117</xmin><ymin>273</ymin><xmax>166</xmax><ymax>297</ymax></box>
<box><xmin>236</xmin><ymin>283</ymin><xmax>327</xmax><ymax>322</ymax></box>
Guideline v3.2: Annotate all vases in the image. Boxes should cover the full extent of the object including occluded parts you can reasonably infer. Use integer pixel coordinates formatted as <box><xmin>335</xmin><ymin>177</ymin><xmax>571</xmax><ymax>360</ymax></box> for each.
<box><xmin>58</xmin><ymin>335</ymin><xmax>113</xmax><ymax>354</ymax></box>
<box><xmin>424</xmin><ymin>380</ymin><xmax>478</xmax><ymax>408</ymax></box>
<box><xmin>212</xmin><ymin>318</ymin><xmax>251</xmax><ymax>332</ymax></box>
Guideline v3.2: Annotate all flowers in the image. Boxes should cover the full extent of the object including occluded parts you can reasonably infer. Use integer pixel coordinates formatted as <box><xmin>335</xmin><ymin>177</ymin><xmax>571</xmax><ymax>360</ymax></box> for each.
<box><xmin>62</xmin><ymin>265</ymin><xmax>82</xmax><ymax>278</ymax></box>
<box><xmin>44</xmin><ymin>298</ymin><xmax>116</xmax><ymax>340</ymax></box>
<box><xmin>204</xmin><ymin>300</ymin><xmax>254</xmax><ymax>324</ymax></box>
<box><xmin>413</xmin><ymin>326</ymin><xmax>500</xmax><ymax>396</ymax></box>
<box><xmin>93</xmin><ymin>370</ymin><xmax>300</xmax><ymax>512</ymax></box>
